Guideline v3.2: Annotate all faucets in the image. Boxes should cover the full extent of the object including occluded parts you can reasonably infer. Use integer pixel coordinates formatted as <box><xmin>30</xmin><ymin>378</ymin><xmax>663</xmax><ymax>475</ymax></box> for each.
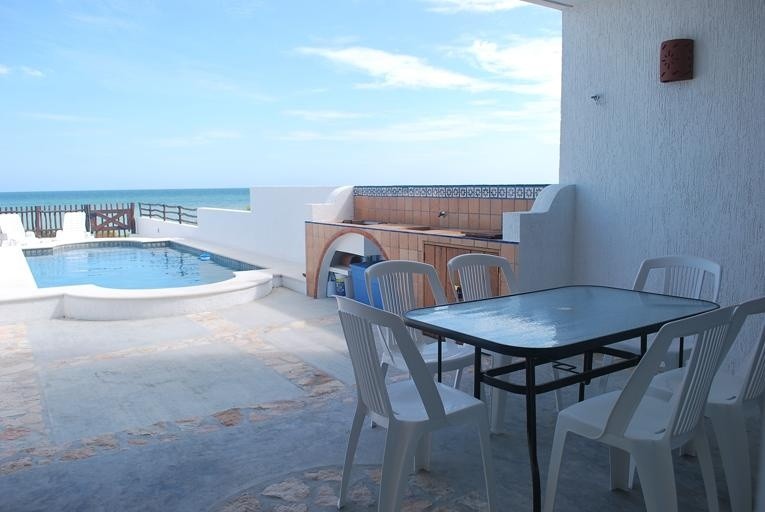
<box><xmin>438</xmin><ymin>210</ymin><xmax>446</xmax><ymax>218</ymax></box>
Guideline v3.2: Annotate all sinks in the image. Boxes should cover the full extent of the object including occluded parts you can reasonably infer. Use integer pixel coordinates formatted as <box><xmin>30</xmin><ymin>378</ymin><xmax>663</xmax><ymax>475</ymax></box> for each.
<box><xmin>396</xmin><ymin>227</ymin><xmax>443</xmax><ymax>232</ymax></box>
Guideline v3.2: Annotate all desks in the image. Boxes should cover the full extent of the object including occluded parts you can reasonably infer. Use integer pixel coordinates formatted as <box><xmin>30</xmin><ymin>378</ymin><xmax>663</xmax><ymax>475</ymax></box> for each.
<box><xmin>402</xmin><ymin>284</ymin><xmax>721</xmax><ymax>510</ymax></box>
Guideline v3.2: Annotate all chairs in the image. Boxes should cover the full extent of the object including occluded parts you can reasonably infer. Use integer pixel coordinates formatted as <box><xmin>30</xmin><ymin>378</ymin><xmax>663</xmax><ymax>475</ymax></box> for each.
<box><xmin>545</xmin><ymin>303</ymin><xmax>737</xmax><ymax>512</ymax></box>
<box><xmin>650</xmin><ymin>296</ymin><xmax>763</xmax><ymax>511</ymax></box>
<box><xmin>600</xmin><ymin>254</ymin><xmax>723</xmax><ymax>393</ymax></box>
<box><xmin>443</xmin><ymin>255</ymin><xmax>566</xmax><ymax>414</ymax></box>
<box><xmin>362</xmin><ymin>259</ymin><xmax>489</xmax><ymax>428</ymax></box>
<box><xmin>331</xmin><ymin>293</ymin><xmax>502</xmax><ymax>512</ymax></box>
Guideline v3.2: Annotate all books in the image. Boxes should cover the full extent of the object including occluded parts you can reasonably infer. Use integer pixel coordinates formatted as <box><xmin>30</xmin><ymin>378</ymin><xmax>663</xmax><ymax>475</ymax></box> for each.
<box><xmin>330</xmin><ymin>264</ymin><xmax>351</xmax><ymax>276</ymax></box>
<box><xmin>460</xmin><ymin>229</ymin><xmax>503</xmax><ymax>239</ymax></box>
<box><xmin>342</xmin><ymin>219</ymin><xmax>367</xmax><ymax>224</ymax></box>
